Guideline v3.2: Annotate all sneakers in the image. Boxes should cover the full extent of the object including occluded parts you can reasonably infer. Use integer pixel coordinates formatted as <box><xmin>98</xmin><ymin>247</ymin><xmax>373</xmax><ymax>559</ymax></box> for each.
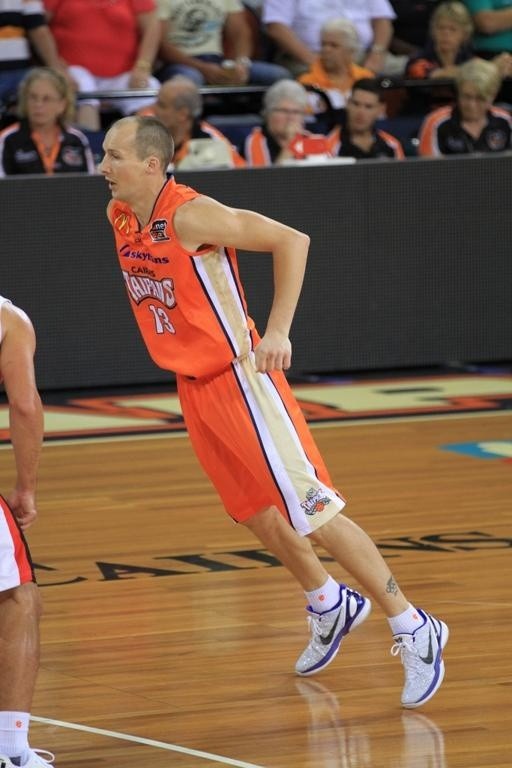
<box><xmin>293</xmin><ymin>582</ymin><xmax>372</xmax><ymax>676</ymax></box>
<box><xmin>391</xmin><ymin>606</ymin><xmax>452</xmax><ymax>708</ymax></box>
<box><xmin>1</xmin><ymin>747</ymin><xmax>54</xmax><ymax>768</ymax></box>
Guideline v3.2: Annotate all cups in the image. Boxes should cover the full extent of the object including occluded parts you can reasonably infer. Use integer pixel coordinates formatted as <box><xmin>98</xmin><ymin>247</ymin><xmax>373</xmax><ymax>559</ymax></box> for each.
<box><xmin>306</xmin><ymin>133</ymin><xmax>327</xmax><ymax>161</ymax></box>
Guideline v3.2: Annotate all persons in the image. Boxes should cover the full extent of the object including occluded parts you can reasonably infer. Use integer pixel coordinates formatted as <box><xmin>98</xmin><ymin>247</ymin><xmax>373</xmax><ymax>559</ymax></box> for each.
<box><xmin>1</xmin><ymin>1</ymin><xmax>511</xmax><ymax>176</ymax></box>
<box><xmin>0</xmin><ymin>296</ymin><xmax>57</xmax><ymax>768</ymax></box>
<box><xmin>102</xmin><ymin>117</ymin><xmax>451</xmax><ymax>708</ymax></box>
